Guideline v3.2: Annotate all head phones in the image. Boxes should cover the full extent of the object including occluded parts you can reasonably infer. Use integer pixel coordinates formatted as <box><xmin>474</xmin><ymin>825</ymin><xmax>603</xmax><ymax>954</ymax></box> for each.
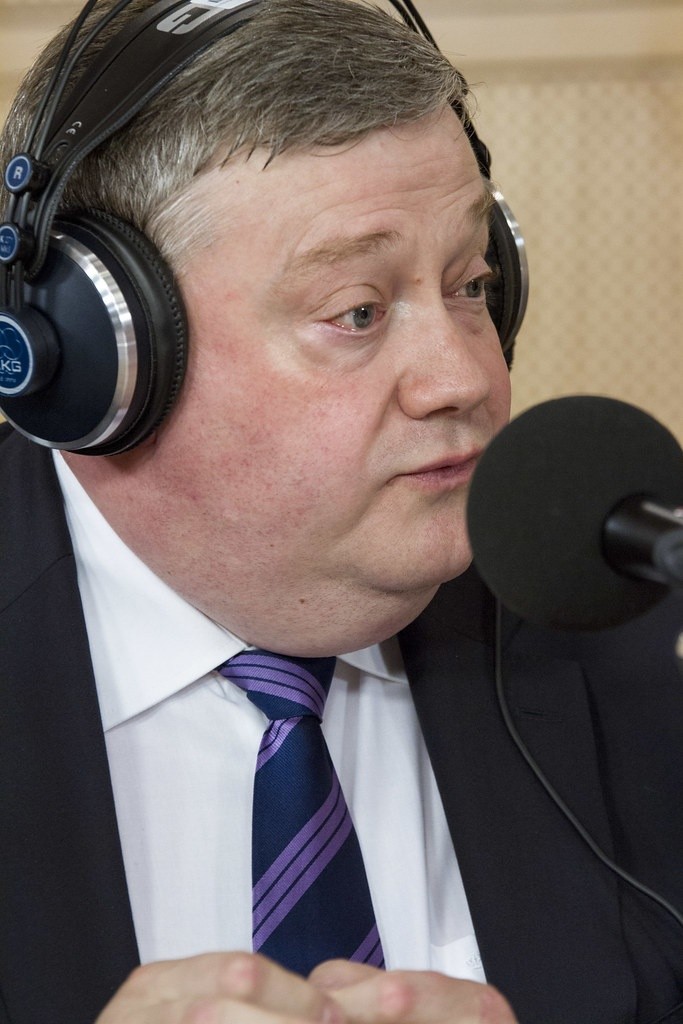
<box><xmin>0</xmin><ymin>0</ymin><xmax>530</xmax><ymax>459</ymax></box>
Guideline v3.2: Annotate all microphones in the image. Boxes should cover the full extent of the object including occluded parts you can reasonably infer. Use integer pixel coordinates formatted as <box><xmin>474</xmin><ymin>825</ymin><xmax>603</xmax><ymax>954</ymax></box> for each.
<box><xmin>467</xmin><ymin>395</ymin><xmax>683</xmax><ymax>626</ymax></box>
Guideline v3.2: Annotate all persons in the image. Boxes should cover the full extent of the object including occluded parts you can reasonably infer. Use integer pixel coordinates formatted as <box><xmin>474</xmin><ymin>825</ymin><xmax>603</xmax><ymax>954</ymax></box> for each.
<box><xmin>0</xmin><ymin>0</ymin><xmax>683</xmax><ymax>1024</ymax></box>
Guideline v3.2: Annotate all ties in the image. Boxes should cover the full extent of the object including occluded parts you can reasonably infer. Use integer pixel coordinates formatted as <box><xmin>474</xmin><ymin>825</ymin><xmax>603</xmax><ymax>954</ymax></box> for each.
<box><xmin>217</xmin><ymin>650</ymin><xmax>387</xmax><ymax>983</ymax></box>
